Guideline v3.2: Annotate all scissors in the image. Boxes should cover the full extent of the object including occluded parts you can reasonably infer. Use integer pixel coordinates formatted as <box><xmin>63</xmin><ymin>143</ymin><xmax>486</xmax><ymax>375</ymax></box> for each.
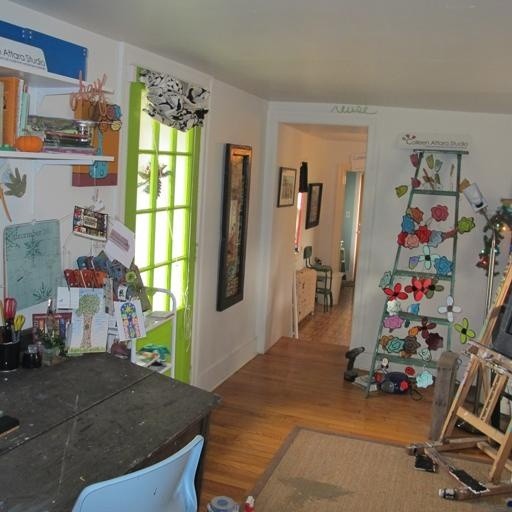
<box><xmin>14</xmin><ymin>313</ymin><xmax>26</xmax><ymax>332</ymax></box>
<box><xmin>3</xmin><ymin>296</ymin><xmax>17</xmax><ymax>327</ymax></box>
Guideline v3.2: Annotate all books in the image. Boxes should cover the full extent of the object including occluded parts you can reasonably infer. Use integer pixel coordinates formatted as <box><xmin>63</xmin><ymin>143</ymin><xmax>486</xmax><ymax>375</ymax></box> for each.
<box><xmin>0</xmin><ymin>408</ymin><xmax>20</xmax><ymax>437</ymax></box>
<box><xmin>0</xmin><ymin>76</ymin><xmax>97</xmax><ymax>157</ymax></box>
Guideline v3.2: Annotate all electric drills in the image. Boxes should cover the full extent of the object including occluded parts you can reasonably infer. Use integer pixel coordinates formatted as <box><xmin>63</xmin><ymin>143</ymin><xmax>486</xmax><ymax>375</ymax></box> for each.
<box><xmin>342</xmin><ymin>346</ymin><xmax>366</xmax><ymax>382</ymax></box>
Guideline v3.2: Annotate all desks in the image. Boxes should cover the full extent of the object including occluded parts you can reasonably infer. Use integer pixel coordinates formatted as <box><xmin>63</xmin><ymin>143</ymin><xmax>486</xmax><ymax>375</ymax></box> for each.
<box><xmin>0</xmin><ymin>352</ymin><xmax>223</xmax><ymax>512</ymax></box>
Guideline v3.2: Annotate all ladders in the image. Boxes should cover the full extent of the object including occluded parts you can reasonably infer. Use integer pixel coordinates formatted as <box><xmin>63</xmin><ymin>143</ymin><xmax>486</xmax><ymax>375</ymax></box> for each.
<box><xmin>366</xmin><ymin>149</ymin><xmax>469</xmax><ymax>399</ymax></box>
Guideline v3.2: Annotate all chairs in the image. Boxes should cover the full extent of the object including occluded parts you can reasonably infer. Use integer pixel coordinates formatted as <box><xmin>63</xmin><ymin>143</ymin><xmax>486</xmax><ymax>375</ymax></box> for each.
<box><xmin>68</xmin><ymin>435</ymin><xmax>204</xmax><ymax>512</ymax></box>
<box><xmin>304</xmin><ymin>245</ymin><xmax>333</xmax><ymax>312</ymax></box>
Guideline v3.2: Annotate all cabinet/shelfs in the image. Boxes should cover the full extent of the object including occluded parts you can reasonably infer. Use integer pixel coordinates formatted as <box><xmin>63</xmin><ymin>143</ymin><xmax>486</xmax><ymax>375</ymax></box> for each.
<box><xmin>0</xmin><ymin>58</ymin><xmax>115</xmax><ymax>165</ymax></box>
<box><xmin>107</xmin><ymin>288</ymin><xmax>176</xmax><ymax>381</ymax></box>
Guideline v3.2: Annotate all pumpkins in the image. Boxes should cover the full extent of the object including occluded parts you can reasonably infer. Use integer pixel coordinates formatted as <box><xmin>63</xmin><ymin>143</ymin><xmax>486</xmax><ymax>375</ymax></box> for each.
<box><xmin>15</xmin><ymin>128</ymin><xmax>44</xmax><ymax>153</ymax></box>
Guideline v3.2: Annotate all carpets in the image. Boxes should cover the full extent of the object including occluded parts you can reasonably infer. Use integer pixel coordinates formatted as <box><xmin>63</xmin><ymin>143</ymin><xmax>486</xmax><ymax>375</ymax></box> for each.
<box><xmin>244</xmin><ymin>424</ymin><xmax>510</xmax><ymax>511</ymax></box>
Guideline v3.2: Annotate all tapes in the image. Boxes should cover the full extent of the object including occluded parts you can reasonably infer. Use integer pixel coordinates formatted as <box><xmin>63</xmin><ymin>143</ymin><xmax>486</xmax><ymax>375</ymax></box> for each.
<box><xmin>210</xmin><ymin>495</ymin><xmax>236</xmax><ymax>512</ymax></box>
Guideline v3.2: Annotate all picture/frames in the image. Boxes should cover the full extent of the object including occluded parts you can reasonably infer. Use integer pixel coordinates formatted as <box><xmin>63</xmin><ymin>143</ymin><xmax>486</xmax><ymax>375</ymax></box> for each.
<box><xmin>305</xmin><ymin>183</ymin><xmax>322</xmax><ymax>229</ymax></box>
<box><xmin>215</xmin><ymin>142</ymin><xmax>252</xmax><ymax>312</ymax></box>
<box><xmin>277</xmin><ymin>166</ymin><xmax>297</xmax><ymax>208</ymax></box>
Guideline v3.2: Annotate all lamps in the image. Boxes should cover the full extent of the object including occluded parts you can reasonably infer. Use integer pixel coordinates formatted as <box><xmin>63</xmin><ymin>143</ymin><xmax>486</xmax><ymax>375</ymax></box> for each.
<box><xmin>455</xmin><ymin>182</ymin><xmax>499</xmax><ymax>437</ymax></box>
